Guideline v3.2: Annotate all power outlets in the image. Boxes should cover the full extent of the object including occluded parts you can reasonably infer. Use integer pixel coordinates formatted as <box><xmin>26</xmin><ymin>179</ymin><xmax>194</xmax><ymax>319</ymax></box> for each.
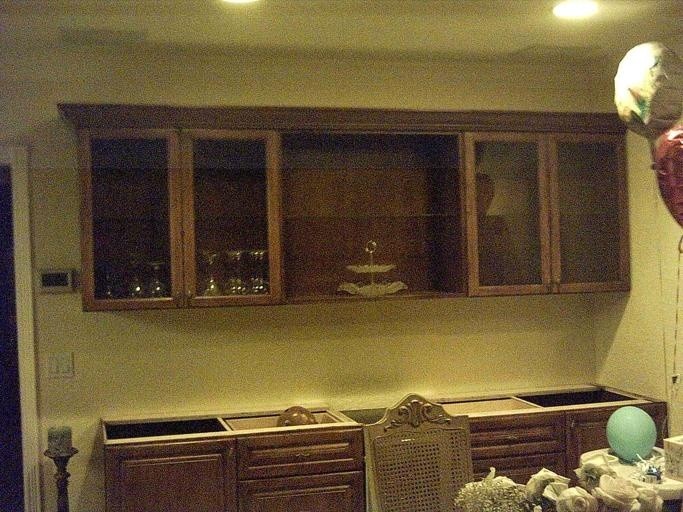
<box><xmin>45</xmin><ymin>348</ymin><xmax>74</xmax><ymax>379</ymax></box>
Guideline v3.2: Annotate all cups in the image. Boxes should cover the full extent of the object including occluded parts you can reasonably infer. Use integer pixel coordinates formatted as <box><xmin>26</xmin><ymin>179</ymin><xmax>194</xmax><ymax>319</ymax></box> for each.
<box><xmin>147</xmin><ymin>260</ymin><xmax>168</xmax><ymax>299</ymax></box>
<box><xmin>197</xmin><ymin>250</ymin><xmax>221</xmax><ymax>295</ymax></box>
<box><xmin>123</xmin><ymin>256</ymin><xmax>146</xmax><ymax>300</ymax></box>
<box><xmin>223</xmin><ymin>249</ymin><xmax>247</xmax><ymax>295</ymax></box>
<box><xmin>249</xmin><ymin>251</ymin><xmax>269</xmax><ymax>295</ymax></box>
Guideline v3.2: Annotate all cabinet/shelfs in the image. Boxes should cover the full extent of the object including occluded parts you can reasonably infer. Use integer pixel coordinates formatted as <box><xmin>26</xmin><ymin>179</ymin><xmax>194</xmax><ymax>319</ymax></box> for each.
<box><xmin>429</xmin><ymin>393</ymin><xmax>568</xmax><ymax>484</ymax></box>
<box><xmin>55</xmin><ymin>102</ymin><xmax>632</xmax><ymax>314</ymax></box>
<box><xmin>222</xmin><ymin>406</ymin><xmax>365</xmax><ymax>512</ymax></box>
<box><xmin>514</xmin><ymin>383</ymin><xmax>671</xmax><ymax>490</ymax></box>
<box><xmin>101</xmin><ymin>415</ymin><xmax>238</xmax><ymax>511</ymax></box>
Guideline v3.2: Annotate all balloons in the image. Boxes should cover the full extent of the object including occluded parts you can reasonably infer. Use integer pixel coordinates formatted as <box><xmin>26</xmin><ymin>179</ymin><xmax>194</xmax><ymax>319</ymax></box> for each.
<box><xmin>615</xmin><ymin>41</ymin><xmax>683</xmax><ymax>136</ymax></box>
<box><xmin>656</xmin><ymin>126</ymin><xmax>683</xmax><ymax>230</ymax></box>
<box><xmin>606</xmin><ymin>405</ymin><xmax>657</xmax><ymax>463</ymax></box>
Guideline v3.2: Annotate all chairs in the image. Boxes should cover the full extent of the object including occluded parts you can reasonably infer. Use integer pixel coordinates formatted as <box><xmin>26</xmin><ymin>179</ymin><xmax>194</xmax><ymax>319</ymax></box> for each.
<box><xmin>364</xmin><ymin>394</ymin><xmax>475</xmax><ymax>512</ymax></box>
